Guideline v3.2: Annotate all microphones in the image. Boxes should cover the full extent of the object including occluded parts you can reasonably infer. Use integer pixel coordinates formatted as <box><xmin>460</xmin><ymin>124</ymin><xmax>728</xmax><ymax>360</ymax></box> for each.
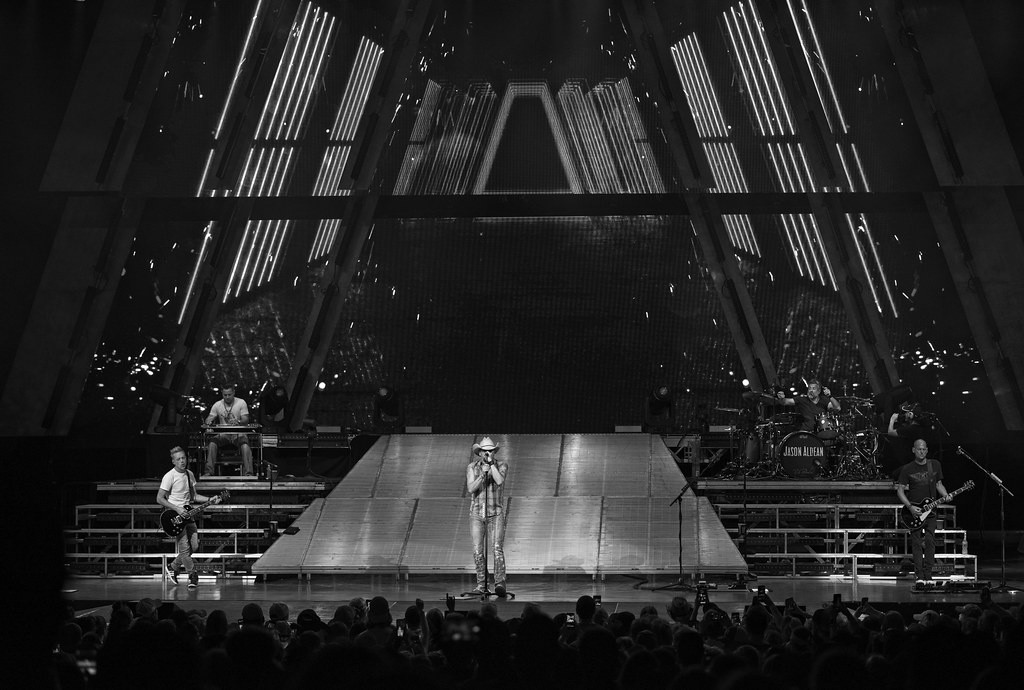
<box><xmin>263</xmin><ymin>460</ymin><xmax>278</xmax><ymax>467</ymax></box>
<box><xmin>485</xmin><ymin>453</ymin><xmax>489</xmax><ymax>457</ymax></box>
<box><xmin>814</xmin><ymin>459</ymin><xmax>823</xmax><ymax>469</ymax></box>
<box><xmin>956</xmin><ymin>446</ymin><xmax>961</xmax><ymax>455</ymax></box>
<box><xmin>707</xmin><ymin>449</ymin><xmax>720</xmax><ymax>461</ymax></box>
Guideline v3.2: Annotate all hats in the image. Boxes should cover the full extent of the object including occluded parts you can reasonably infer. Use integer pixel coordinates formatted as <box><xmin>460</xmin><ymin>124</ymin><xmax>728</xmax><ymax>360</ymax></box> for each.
<box><xmin>242</xmin><ymin>603</ymin><xmax>265</xmax><ymax>624</ymax></box>
<box><xmin>135</xmin><ymin>598</ymin><xmax>161</xmax><ymax>617</ymax></box>
<box><xmin>368</xmin><ymin>597</ymin><xmax>389</xmax><ymax>619</ymax></box>
<box><xmin>651</xmin><ymin>616</ymin><xmax>683</xmax><ymax>641</ymax></box>
<box><xmin>955</xmin><ymin>604</ymin><xmax>982</xmax><ymax>616</ymax></box>
<box><xmin>473</xmin><ymin>437</ymin><xmax>500</xmax><ymax>457</ymax></box>
<box><xmin>913</xmin><ymin>610</ymin><xmax>939</xmax><ymax>624</ymax></box>
<box><xmin>269</xmin><ymin>603</ymin><xmax>289</xmax><ymax>621</ymax></box>
<box><xmin>297</xmin><ymin>609</ymin><xmax>321</xmax><ymax>628</ymax></box>
<box><xmin>666</xmin><ymin>597</ymin><xmax>694</xmax><ymax>620</ymax></box>
<box><xmin>185</xmin><ymin>609</ymin><xmax>207</xmax><ymax>618</ymax></box>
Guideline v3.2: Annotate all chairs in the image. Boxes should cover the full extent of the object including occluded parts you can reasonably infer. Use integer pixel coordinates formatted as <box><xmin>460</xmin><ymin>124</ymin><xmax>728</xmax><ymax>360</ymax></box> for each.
<box><xmin>188</xmin><ymin>433</ymin><xmax>278</xmax><ymax>476</ymax></box>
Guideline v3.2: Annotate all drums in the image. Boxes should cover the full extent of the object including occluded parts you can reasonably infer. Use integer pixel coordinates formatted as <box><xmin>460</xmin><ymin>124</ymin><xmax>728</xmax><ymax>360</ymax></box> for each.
<box><xmin>773</xmin><ymin>412</ymin><xmax>802</xmax><ymax>431</ymax></box>
<box><xmin>775</xmin><ymin>429</ymin><xmax>829</xmax><ymax>478</ymax></box>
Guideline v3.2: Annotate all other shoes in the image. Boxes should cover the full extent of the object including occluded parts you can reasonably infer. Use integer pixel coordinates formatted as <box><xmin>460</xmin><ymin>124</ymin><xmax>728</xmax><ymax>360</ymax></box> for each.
<box><xmin>495</xmin><ymin>584</ymin><xmax>508</xmax><ymax>598</ymax></box>
<box><xmin>472</xmin><ymin>585</ymin><xmax>492</xmax><ymax>593</ymax></box>
<box><xmin>916</xmin><ymin>580</ymin><xmax>935</xmax><ymax>587</ymax></box>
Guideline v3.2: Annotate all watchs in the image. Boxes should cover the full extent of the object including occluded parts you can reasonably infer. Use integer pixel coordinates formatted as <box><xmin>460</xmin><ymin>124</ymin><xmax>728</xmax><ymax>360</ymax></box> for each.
<box><xmin>237</xmin><ymin>419</ymin><xmax>241</xmax><ymax>425</ymax></box>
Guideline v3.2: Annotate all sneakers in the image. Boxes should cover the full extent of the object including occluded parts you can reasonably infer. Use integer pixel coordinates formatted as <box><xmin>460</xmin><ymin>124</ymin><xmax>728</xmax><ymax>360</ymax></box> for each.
<box><xmin>165</xmin><ymin>563</ymin><xmax>178</xmax><ymax>584</ymax></box>
<box><xmin>187</xmin><ymin>572</ymin><xmax>198</xmax><ymax>587</ymax></box>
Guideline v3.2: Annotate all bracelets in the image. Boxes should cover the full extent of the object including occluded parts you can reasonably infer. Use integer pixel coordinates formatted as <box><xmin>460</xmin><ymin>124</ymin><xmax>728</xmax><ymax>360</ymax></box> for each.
<box><xmin>208</xmin><ymin>497</ymin><xmax>211</xmax><ymax>501</ymax></box>
<box><xmin>827</xmin><ymin>395</ymin><xmax>833</xmax><ymax>399</ymax></box>
<box><xmin>907</xmin><ymin>504</ymin><xmax>912</xmax><ymax>510</ymax></box>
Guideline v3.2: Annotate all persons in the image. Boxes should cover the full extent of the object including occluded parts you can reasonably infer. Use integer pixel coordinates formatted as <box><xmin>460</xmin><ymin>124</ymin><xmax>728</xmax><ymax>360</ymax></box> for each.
<box><xmin>0</xmin><ymin>571</ymin><xmax>1024</xmax><ymax>690</ymax></box>
<box><xmin>897</xmin><ymin>439</ymin><xmax>952</xmax><ymax>587</ymax></box>
<box><xmin>204</xmin><ymin>384</ymin><xmax>253</xmax><ymax>478</ymax></box>
<box><xmin>888</xmin><ymin>404</ymin><xmax>927</xmax><ymax>466</ymax></box>
<box><xmin>777</xmin><ymin>379</ymin><xmax>841</xmax><ymax>432</ymax></box>
<box><xmin>466</xmin><ymin>437</ymin><xmax>508</xmax><ymax>598</ymax></box>
<box><xmin>156</xmin><ymin>447</ymin><xmax>222</xmax><ymax>587</ymax></box>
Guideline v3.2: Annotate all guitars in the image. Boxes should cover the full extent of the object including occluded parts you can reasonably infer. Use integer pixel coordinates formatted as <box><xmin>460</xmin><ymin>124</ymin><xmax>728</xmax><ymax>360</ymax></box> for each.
<box><xmin>899</xmin><ymin>478</ymin><xmax>975</xmax><ymax>531</ymax></box>
<box><xmin>158</xmin><ymin>490</ymin><xmax>231</xmax><ymax>540</ymax></box>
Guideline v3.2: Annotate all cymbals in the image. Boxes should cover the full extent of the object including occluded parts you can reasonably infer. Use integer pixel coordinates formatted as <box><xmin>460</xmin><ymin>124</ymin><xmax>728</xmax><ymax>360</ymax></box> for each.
<box><xmin>742</xmin><ymin>391</ymin><xmax>779</xmax><ymax>407</ymax></box>
<box><xmin>835</xmin><ymin>395</ymin><xmax>861</xmax><ymax>401</ymax></box>
<box><xmin>714</xmin><ymin>406</ymin><xmax>739</xmax><ymax>412</ymax></box>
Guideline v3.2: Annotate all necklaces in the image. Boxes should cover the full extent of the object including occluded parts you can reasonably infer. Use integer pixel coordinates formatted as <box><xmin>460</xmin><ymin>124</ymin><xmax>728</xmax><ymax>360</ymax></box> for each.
<box><xmin>223</xmin><ymin>400</ymin><xmax>234</xmax><ymax>416</ymax></box>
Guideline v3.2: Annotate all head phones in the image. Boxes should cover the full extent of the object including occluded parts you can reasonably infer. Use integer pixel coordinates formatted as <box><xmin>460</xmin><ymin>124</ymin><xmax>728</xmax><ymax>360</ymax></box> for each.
<box><xmin>905</xmin><ymin>404</ymin><xmax>917</xmax><ymax>420</ymax></box>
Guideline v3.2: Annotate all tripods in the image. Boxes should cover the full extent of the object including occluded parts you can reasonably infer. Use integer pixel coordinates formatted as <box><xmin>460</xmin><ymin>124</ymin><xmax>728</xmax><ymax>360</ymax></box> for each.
<box><xmin>960</xmin><ymin>450</ymin><xmax>1024</xmax><ymax>592</ymax></box>
<box><xmin>459</xmin><ymin>455</ymin><xmax>515</xmax><ymax>602</ymax></box>
<box><xmin>719</xmin><ymin>344</ymin><xmax>790</xmax><ymax>480</ymax></box>
<box><xmin>652</xmin><ymin>456</ymin><xmax>716</xmax><ymax>592</ymax></box>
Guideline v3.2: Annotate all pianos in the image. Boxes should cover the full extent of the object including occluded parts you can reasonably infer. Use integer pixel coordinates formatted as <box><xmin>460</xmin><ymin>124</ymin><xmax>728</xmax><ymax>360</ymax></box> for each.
<box><xmin>199</xmin><ymin>422</ymin><xmax>264</xmax><ymax>435</ymax></box>
